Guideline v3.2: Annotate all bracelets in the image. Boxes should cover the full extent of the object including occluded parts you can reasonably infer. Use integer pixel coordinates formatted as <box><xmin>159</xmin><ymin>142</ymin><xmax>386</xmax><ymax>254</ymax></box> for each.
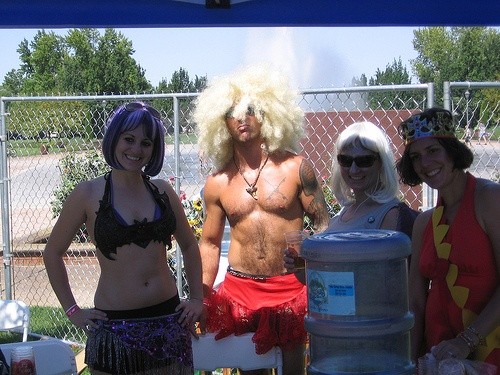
<box><xmin>64</xmin><ymin>303</ymin><xmax>80</xmax><ymax>320</ymax></box>
<box><xmin>456</xmin><ymin>327</ymin><xmax>487</xmax><ymax>354</ymax></box>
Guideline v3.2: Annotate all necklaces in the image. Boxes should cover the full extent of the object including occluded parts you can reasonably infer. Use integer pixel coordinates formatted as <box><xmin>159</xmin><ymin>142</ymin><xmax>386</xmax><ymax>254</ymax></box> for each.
<box><xmin>232</xmin><ymin>151</ymin><xmax>274</xmax><ymax>201</ymax></box>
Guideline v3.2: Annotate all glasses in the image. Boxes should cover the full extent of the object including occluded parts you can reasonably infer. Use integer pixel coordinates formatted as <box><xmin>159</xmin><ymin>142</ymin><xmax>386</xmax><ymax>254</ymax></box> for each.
<box><xmin>124</xmin><ymin>102</ymin><xmax>161</xmax><ymax>121</ymax></box>
<box><xmin>336</xmin><ymin>154</ymin><xmax>379</xmax><ymax>168</ymax></box>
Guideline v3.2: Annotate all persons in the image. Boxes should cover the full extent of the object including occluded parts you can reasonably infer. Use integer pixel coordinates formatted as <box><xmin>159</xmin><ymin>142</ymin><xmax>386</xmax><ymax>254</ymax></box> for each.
<box><xmin>284</xmin><ymin>120</ymin><xmax>423</xmax><ymax>375</ymax></box>
<box><xmin>463</xmin><ymin>120</ymin><xmax>487</xmax><ymax>147</ymax></box>
<box><xmin>188</xmin><ymin>72</ymin><xmax>330</xmax><ymax>375</ymax></box>
<box><xmin>42</xmin><ymin>101</ymin><xmax>203</xmax><ymax>375</ymax></box>
<box><xmin>397</xmin><ymin>107</ymin><xmax>500</xmax><ymax>375</ymax></box>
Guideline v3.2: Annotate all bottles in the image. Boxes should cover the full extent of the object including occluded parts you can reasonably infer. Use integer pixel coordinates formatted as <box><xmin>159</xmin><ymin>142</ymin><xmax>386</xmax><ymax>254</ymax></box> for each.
<box><xmin>301</xmin><ymin>229</ymin><xmax>416</xmax><ymax>375</ymax></box>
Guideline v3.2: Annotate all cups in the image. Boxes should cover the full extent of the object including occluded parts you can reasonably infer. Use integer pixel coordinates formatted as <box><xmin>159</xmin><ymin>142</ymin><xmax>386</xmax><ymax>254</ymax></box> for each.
<box><xmin>418</xmin><ymin>357</ymin><xmax>465</xmax><ymax>375</ymax></box>
<box><xmin>285</xmin><ymin>230</ymin><xmax>307</xmax><ymax>270</ymax></box>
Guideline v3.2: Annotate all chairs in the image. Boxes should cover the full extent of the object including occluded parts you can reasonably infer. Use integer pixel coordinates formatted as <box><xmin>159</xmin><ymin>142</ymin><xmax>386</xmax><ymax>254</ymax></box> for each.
<box><xmin>191</xmin><ymin>331</ymin><xmax>282</xmax><ymax>375</ymax></box>
<box><xmin>0</xmin><ymin>340</ymin><xmax>77</xmax><ymax>375</ymax></box>
<box><xmin>1</xmin><ymin>299</ymin><xmax>30</xmax><ymax>343</ymax></box>
